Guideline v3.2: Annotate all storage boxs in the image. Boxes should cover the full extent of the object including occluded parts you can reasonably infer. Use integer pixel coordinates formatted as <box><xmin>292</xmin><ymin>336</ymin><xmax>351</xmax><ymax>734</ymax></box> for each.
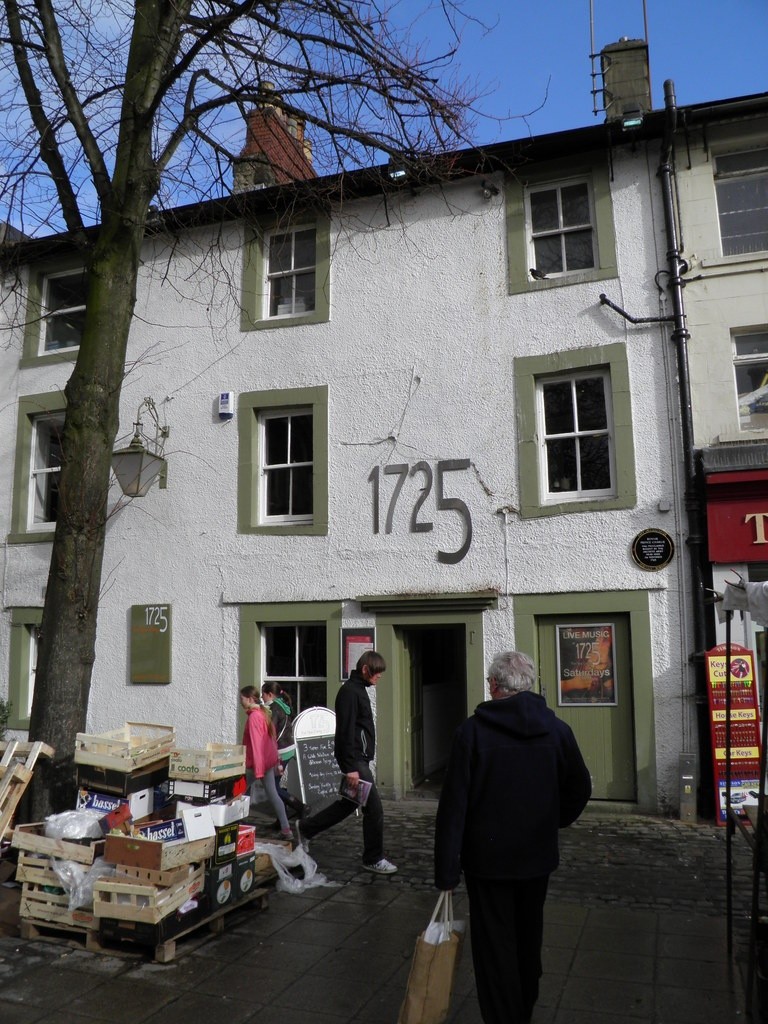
<box><xmin>11</xmin><ymin>721</ymin><xmax>291</xmax><ymax>942</ymax></box>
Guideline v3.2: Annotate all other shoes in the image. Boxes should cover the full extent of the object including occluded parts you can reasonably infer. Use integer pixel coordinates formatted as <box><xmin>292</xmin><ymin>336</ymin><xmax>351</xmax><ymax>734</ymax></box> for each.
<box><xmin>272</xmin><ymin>830</ymin><xmax>293</xmax><ymax>841</ymax></box>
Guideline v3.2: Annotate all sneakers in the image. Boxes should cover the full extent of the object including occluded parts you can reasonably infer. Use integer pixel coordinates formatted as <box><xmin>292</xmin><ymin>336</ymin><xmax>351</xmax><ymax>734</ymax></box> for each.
<box><xmin>362</xmin><ymin>858</ymin><xmax>398</xmax><ymax>874</ymax></box>
<box><xmin>295</xmin><ymin>819</ymin><xmax>310</xmax><ymax>852</ymax></box>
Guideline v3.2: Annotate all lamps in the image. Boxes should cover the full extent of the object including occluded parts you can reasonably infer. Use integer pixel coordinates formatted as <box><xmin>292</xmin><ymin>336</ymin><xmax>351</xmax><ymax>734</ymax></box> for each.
<box><xmin>145</xmin><ymin>204</ymin><xmax>165</xmax><ymax>227</ymax></box>
<box><xmin>621</xmin><ymin>115</ymin><xmax>645</xmax><ymax>130</ymax></box>
<box><xmin>388</xmin><ymin>156</ymin><xmax>421</xmax><ymax>182</ymax></box>
<box><xmin>112</xmin><ymin>397</ymin><xmax>170</xmax><ymax>498</ymax></box>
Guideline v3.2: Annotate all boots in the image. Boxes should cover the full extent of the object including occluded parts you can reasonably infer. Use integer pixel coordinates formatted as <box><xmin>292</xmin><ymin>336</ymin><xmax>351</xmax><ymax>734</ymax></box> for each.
<box><xmin>271</xmin><ymin>802</ymin><xmax>286</xmax><ymax>829</ymax></box>
<box><xmin>286</xmin><ymin>795</ymin><xmax>313</xmax><ymax>821</ymax></box>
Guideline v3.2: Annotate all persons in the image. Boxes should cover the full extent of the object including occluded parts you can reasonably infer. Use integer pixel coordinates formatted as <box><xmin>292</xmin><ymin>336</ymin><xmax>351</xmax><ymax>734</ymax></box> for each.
<box><xmin>296</xmin><ymin>651</ymin><xmax>398</xmax><ymax>874</ymax></box>
<box><xmin>261</xmin><ymin>681</ymin><xmax>313</xmax><ymax>830</ymax></box>
<box><xmin>561</xmin><ymin>626</ymin><xmax>614</xmax><ymax>703</ymax></box>
<box><xmin>239</xmin><ymin>686</ymin><xmax>294</xmax><ymax>840</ymax></box>
<box><xmin>434</xmin><ymin>652</ymin><xmax>591</xmax><ymax>1024</ymax></box>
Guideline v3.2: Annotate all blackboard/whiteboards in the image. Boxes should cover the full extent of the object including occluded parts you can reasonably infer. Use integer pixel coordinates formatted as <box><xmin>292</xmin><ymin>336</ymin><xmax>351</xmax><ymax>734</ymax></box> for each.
<box><xmin>296</xmin><ymin>734</ymin><xmax>341</xmax><ymax>815</ymax></box>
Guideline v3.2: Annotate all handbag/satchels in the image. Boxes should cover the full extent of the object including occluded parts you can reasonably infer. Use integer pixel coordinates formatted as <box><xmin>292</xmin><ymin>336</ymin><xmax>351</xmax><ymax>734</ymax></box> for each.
<box><xmin>395</xmin><ymin>892</ymin><xmax>462</xmax><ymax>1024</ymax></box>
<box><xmin>249</xmin><ymin>778</ymin><xmax>269</xmax><ymax>806</ymax></box>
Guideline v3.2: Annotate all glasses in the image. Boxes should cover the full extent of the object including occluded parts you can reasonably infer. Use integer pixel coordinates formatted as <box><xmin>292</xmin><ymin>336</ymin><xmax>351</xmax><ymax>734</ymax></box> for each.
<box><xmin>486</xmin><ymin>677</ymin><xmax>491</xmax><ymax>682</ymax></box>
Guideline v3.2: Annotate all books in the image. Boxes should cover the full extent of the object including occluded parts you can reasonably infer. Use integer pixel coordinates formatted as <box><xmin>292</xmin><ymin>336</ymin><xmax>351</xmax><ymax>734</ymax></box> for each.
<box><xmin>337</xmin><ymin>772</ymin><xmax>372</xmax><ymax>807</ymax></box>
